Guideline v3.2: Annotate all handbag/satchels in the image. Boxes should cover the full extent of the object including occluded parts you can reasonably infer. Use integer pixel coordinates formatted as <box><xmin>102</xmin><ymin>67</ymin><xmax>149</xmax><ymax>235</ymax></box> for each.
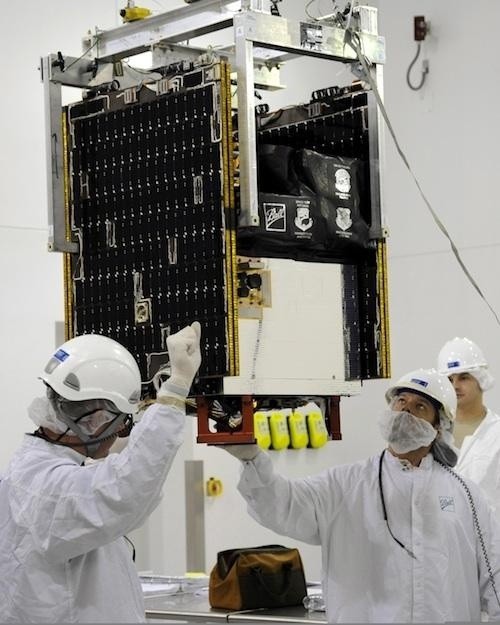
<box><xmin>208</xmin><ymin>544</ymin><xmax>309</xmax><ymax>609</ymax></box>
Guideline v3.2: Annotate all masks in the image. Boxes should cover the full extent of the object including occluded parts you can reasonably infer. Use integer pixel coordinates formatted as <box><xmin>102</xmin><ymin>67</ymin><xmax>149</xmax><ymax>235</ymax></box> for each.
<box><xmin>376</xmin><ymin>409</ymin><xmax>439</xmax><ymax>456</ymax></box>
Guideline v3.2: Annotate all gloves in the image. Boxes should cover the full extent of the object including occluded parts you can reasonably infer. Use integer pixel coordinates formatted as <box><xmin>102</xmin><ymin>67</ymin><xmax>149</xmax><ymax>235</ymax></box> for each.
<box><xmin>213</xmin><ymin>443</ymin><xmax>259</xmax><ymax>462</ymax></box>
<box><xmin>157</xmin><ymin>320</ymin><xmax>203</xmax><ymax>398</ymax></box>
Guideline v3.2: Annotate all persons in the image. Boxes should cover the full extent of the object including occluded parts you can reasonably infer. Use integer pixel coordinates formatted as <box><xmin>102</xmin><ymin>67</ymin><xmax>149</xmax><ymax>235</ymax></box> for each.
<box><xmin>0</xmin><ymin>315</ymin><xmax>203</xmax><ymax>624</ymax></box>
<box><xmin>212</xmin><ymin>366</ymin><xmax>500</xmax><ymax>625</ymax></box>
<box><xmin>432</xmin><ymin>333</ymin><xmax>500</xmax><ymax>624</ymax></box>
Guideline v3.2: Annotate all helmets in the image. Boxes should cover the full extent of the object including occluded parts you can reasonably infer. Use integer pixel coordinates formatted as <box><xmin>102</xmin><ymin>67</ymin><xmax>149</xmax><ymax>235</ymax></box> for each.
<box><xmin>37</xmin><ymin>332</ymin><xmax>142</xmax><ymax>414</ymax></box>
<box><xmin>436</xmin><ymin>336</ymin><xmax>488</xmax><ymax>374</ymax></box>
<box><xmin>383</xmin><ymin>367</ymin><xmax>457</xmax><ymax>423</ymax></box>
